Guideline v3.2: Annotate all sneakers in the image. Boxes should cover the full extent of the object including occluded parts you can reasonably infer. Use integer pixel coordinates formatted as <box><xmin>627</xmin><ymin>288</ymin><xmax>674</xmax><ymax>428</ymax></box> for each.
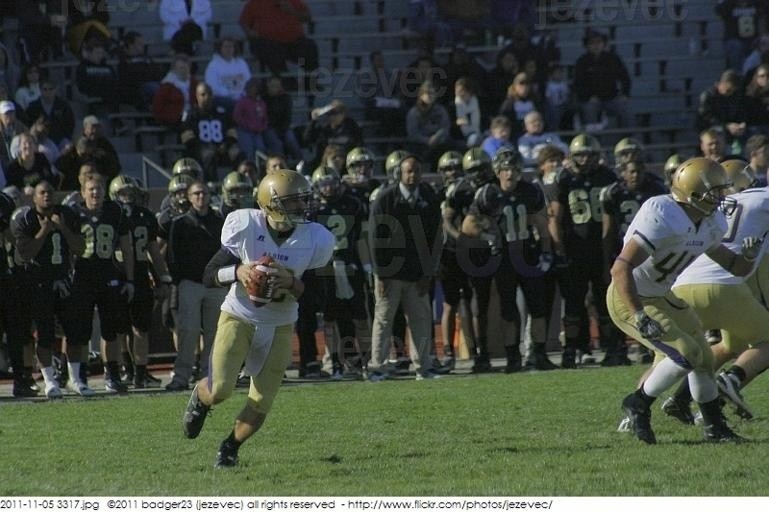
<box><xmin>661</xmin><ymin>397</ymin><xmax>694</xmax><ymax>425</ymax></box>
<box><xmin>427</xmin><ymin>359</ymin><xmax>450</xmax><ymax>375</ymax></box>
<box><xmin>561</xmin><ymin>353</ymin><xmax>576</xmax><ymax>369</ymax></box>
<box><xmin>12</xmin><ymin>386</ymin><xmax>37</xmax><ymax>397</ymax></box>
<box><xmin>165</xmin><ymin>381</ymin><xmax>186</xmax><ymax>391</ymax></box>
<box><xmin>23</xmin><ymin>377</ymin><xmax>39</xmax><ymax>390</ymax></box>
<box><xmin>608</xmin><ymin>351</ymin><xmax>630</xmax><ymax>364</ymax></box>
<box><xmin>501</xmin><ymin>353</ymin><xmax>522</xmax><ymax>372</ymax></box>
<box><xmin>529</xmin><ymin>351</ymin><xmax>559</xmax><ymax>370</ymax></box>
<box><xmin>183</xmin><ymin>386</ymin><xmax>210</xmax><ymax>439</ymax></box>
<box><xmin>579</xmin><ymin>353</ymin><xmax>594</xmax><ymax>363</ymax></box>
<box><xmin>305</xmin><ymin>366</ymin><xmax>326</xmax><ymax>378</ymax></box>
<box><xmin>396</xmin><ymin>358</ymin><xmax>416</xmax><ymax>371</ymax></box>
<box><xmin>370</xmin><ymin>368</ymin><xmax>390</xmax><ymax>380</ymax></box>
<box><xmin>66</xmin><ymin>380</ymin><xmax>94</xmax><ymax>396</ymax></box>
<box><xmin>343</xmin><ymin>369</ymin><xmax>365</xmax><ymax>380</ymax></box>
<box><xmin>716</xmin><ymin>372</ymin><xmax>754</xmax><ymax>419</ymax></box>
<box><xmin>442</xmin><ymin>354</ymin><xmax>455</xmax><ymax>368</ymax></box>
<box><xmin>214</xmin><ymin>439</ymin><xmax>238</xmax><ymax>468</ymax></box>
<box><xmin>45</xmin><ymin>385</ymin><xmax>62</xmax><ymax>398</ymax></box>
<box><xmin>415</xmin><ymin>369</ymin><xmax>440</xmax><ymax>379</ymax></box>
<box><xmin>105</xmin><ymin>379</ymin><xmax>128</xmax><ymax>393</ymax></box>
<box><xmin>330</xmin><ymin>365</ymin><xmax>344</xmax><ymax>379</ymax></box>
<box><xmin>473</xmin><ymin>358</ymin><xmax>490</xmax><ymax>372</ymax></box>
<box><xmin>121</xmin><ymin>374</ymin><xmax>133</xmax><ymax>385</ymax></box>
<box><xmin>621</xmin><ymin>393</ymin><xmax>656</xmax><ymax>445</ymax></box>
<box><xmin>133</xmin><ymin>374</ymin><xmax>161</xmax><ymax>389</ymax></box>
<box><xmin>703</xmin><ymin>422</ymin><xmax>750</xmax><ymax>443</ymax></box>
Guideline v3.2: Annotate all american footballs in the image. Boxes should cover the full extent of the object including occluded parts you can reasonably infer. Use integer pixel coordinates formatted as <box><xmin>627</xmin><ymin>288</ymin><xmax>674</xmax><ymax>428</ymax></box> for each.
<box><xmin>248</xmin><ymin>257</ymin><xmax>280</xmax><ymax>309</ymax></box>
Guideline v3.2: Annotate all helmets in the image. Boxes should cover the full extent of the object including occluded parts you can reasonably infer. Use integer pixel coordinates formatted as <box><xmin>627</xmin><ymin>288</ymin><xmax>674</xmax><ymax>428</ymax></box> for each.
<box><xmin>313</xmin><ymin>166</ymin><xmax>340</xmax><ymax>198</ymax></box>
<box><xmin>463</xmin><ymin>149</ymin><xmax>490</xmax><ymax>174</ymax></box>
<box><xmin>347</xmin><ymin>149</ymin><xmax>376</xmax><ymax>179</ymax></box>
<box><xmin>109</xmin><ymin>176</ymin><xmax>138</xmax><ymax>205</ymax></box>
<box><xmin>135</xmin><ymin>178</ymin><xmax>150</xmax><ymax>208</ymax></box>
<box><xmin>173</xmin><ymin>158</ymin><xmax>203</xmax><ymax>182</ymax></box>
<box><xmin>222</xmin><ymin>173</ymin><xmax>253</xmax><ymax>208</ymax></box>
<box><xmin>570</xmin><ymin>135</ymin><xmax>600</xmax><ymax>165</ymax></box>
<box><xmin>438</xmin><ymin>152</ymin><xmax>462</xmax><ymax>178</ymax></box>
<box><xmin>716</xmin><ymin>160</ymin><xmax>760</xmax><ymax>200</ymax></box>
<box><xmin>492</xmin><ymin>148</ymin><xmax>523</xmax><ymax>180</ymax></box>
<box><xmin>385</xmin><ymin>150</ymin><xmax>411</xmax><ymax>180</ymax></box>
<box><xmin>614</xmin><ymin>139</ymin><xmax>642</xmax><ymax>155</ymax></box>
<box><xmin>168</xmin><ymin>174</ymin><xmax>195</xmax><ymax>206</ymax></box>
<box><xmin>258</xmin><ymin>171</ymin><xmax>311</xmax><ymax>224</ymax></box>
<box><xmin>672</xmin><ymin>158</ymin><xmax>734</xmax><ymax>215</ymax></box>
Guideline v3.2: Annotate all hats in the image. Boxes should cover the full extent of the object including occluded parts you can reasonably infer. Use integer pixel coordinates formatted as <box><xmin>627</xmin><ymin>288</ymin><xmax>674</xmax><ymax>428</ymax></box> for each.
<box><xmin>0</xmin><ymin>100</ymin><xmax>15</xmax><ymax>114</ymax></box>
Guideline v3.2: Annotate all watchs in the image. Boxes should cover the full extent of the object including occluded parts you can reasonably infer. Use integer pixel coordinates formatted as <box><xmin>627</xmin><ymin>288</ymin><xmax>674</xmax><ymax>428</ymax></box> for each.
<box><xmin>126</xmin><ymin>278</ymin><xmax>138</xmax><ymax>286</ymax></box>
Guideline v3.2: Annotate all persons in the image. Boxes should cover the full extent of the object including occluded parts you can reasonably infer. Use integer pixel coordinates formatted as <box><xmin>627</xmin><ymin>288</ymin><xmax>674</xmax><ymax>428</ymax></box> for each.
<box><xmin>110</xmin><ymin>175</ymin><xmax>172</xmax><ymax>391</ymax></box>
<box><xmin>719</xmin><ymin>160</ymin><xmax>761</xmax><ymax>195</ymax></box>
<box><xmin>233</xmin><ymin>78</ymin><xmax>267</xmax><ymax>162</ymax></box>
<box><xmin>297</xmin><ymin>167</ymin><xmax>368</xmax><ymax>378</ymax></box>
<box><xmin>265</xmin><ymin>155</ymin><xmax>288</xmax><ymax>171</ymax></box>
<box><xmin>441</xmin><ymin>146</ymin><xmax>501</xmax><ymax>369</ymax></box>
<box><xmin>1</xmin><ymin>190</ymin><xmax>18</xmax><ymax>379</ymax></box>
<box><xmin>413</xmin><ymin>43</ymin><xmax>483</xmax><ymax>83</ymax></box>
<box><xmin>361</xmin><ymin>53</ymin><xmax>404</xmax><ymax>132</ymax></box>
<box><xmin>747</xmin><ymin>136</ymin><xmax>769</xmax><ymax>184</ymax></box>
<box><xmin>216</xmin><ymin>172</ymin><xmax>257</xmax><ymax>214</ymax></box>
<box><xmin>183</xmin><ymin>171</ymin><xmax>338</xmax><ymax>471</ymax></box>
<box><xmin>56</xmin><ymin>137</ymin><xmax>98</xmax><ymax>172</ymax></box>
<box><xmin>548</xmin><ymin>135</ymin><xmax>617</xmax><ymax>367</ymax></box>
<box><xmin>717</xmin><ymin>0</ymin><xmax>769</xmax><ymax>70</ymax></box>
<box><xmin>461</xmin><ymin>147</ymin><xmax>557</xmax><ymax>372</ymax></box>
<box><xmin>10</xmin><ymin>117</ymin><xmax>62</xmax><ymax>164</ymax></box>
<box><xmin>544</xmin><ymin>65</ymin><xmax>568</xmax><ymax>107</ymax></box>
<box><xmin>261</xmin><ymin>75</ymin><xmax>301</xmax><ymax>159</ymax></box>
<box><xmin>178</xmin><ymin>82</ymin><xmax>248</xmax><ymax>181</ymax></box>
<box><xmin>154</xmin><ymin>53</ymin><xmax>199</xmax><ymax>124</ymax></box>
<box><xmin>5</xmin><ymin>131</ymin><xmax>52</xmax><ymax>185</ymax></box>
<box><xmin>604</xmin><ymin>159</ymin><xmax>669</xmax><ymax>359</ymax></box>
<box><xmin>748</xmin><ymin>68</ymin><xmax>768</xmax><ymax>101</ymax></box>
<box><xmin>606</xmin><ymin>158</ymin><xmax>765</xmax><ymax>447</ymax></box>
<box><xmin>406</xmin><ymin>86</ymin><xmax>452</xmax><ymax>156</ymax></box>
<box><xmin>173</xmin><ymin>159</ymin><xmax>204</xmax><ymax>185</ymax></box>
<box><xmin>10</xmin><ymin>181</ymin><xmax>96</xmax><ymax>397</ymax></box>
<box><xmin>69</xmin><ymin>170</ymin><xmax>134</xmax><ymax>392</ymax></box>
<box><xmin>78</xmin><ymin>29</ymin><xmax>158</xmax><ymax>110</ymax></box>
<box><xmin>167</xmin><ymin>184</ymin><xmax>230</xmax><ymax>391</ymax></box>
<box><xmin>324</xmin><ymin>145</ymin><xmax>345</xmax><ymax>175</ymax></box>
<box><xmin>569</xmin><ymin>28</ymin><xmax>632</xmax><ymax>101</ymax></box>
<box><xmin>448</xmin><ymin>80</ymin><xmax>489</xmax><ymax>147</ymax></box>
<box><xmin>600</xmin><ymin>136</ymin><xmax>664</xmax><ymax>236</ymax></box>
<box><xmin>430</xmin><ymin>151</ymin><xmax>466</xmax><ymax>371</ymax></box>
<box><xmin>204</xmin><ymin>38</ymin><xmax>251</xmax><ymax>114</ymax></box>
<box><xmin>61</xmin><ymin>165</ymin><xmax>97</xmax><ymax>202</ymax></box>
<box><xmin>0</xmin><ymin>100</ymin><xmax>16</xmax><ymax>128</ymax></box>
<box><xmin>238</xmin><ymin>164</ymin><xmax>259</xmax><ymax>186</ymax></box>
<box><xmin>322</xmin><ymin>146</ymin><xmax>387</xmax><ymax>376</ymax></box>
<box><xmin>617</xmin><ymin>184</ymin><xmax>769</xmax><ymax>434</ymax></box>
<box><xmin>159</xmin><ymin>0</ymin><xmax>212</xmax><ymax>51</ymax></box>
<box><xmin>695</xmin><ymin>71</ymin><xmax>756</xmax><ymax>134</ymax></box>
<box><xmin>568</xmin><ymin>94</ymin><xmax>611</xmax><ymax>134</ymax></box>
<box><xmin>536</xmin><ymin>145</ymin><xmax>565</xmax><ymax>187</ymax></box>
<box><xmin>240</xmin><ymin>0</ymin><xmax>320</xmax><ymax>91</ymax></box>
<box><xmin>307</xmin><ymin>100</ymin><xmax>360</xmax><ymax>160</ymax></box>
<box><xmin>501</xmin><ymin>73</ymin><xmax>541</xmax><ymax>137</ymax></box>
<box><xmin>482</xmin><ymin>117</ymin><xmax>512</xmax><ymax>158</ymax></box>
<box><xmin>158</xmin><ymin>174</ymin><xmax>193</xmax><ymax>226</ymax></box>
<box><xmin>494</xmin><ymin>22</ymin><xmax>548</xmax><ymax>72</ymax></box>
<box><xmin>516</xmin><ymin>112</ymin><xmax>562</xmax><ymax>165</ymax></box>
<box><xmin>16</xmin><ymin>63</ymin><xmax>42</xmax><ymax>108</ymax></box>
<box><xmin>80</xmin><ymin>115</ymin><xmax>102</xmax><ymax>137</ymax></box>
<box><xmin>695</xmin><ymin>130</ymin><xmax>724</xmax><ymax>160</ymax></box>
<box><xmin>24</xmin><ymin>78</ymin><xmax>73</xmax><ymax>137</ymax></box>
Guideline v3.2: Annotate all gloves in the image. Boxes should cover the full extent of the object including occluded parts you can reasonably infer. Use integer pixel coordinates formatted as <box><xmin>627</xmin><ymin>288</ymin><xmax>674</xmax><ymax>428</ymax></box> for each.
<box><xmin>537</xmin><ymin>253</ymin><xmax>554</xmax><ymax>271</ymax></box>
<box><xmin>741</xmin><ymin>236</ymin><xmax>764</xmax><ymax>259</ymax></box>
<box><xmin>121</xmin><ymin>282</ymin><xmax>136</xmax><ymax>304</ymax></box>
<box><xmin>635</xmin><ymin>313</ymin><xmax>665</xmax><ymax>339</ymax></box>
<box><xmin>487</xmin><ymin>237</ymin><xmax>503</xmax><ymax>256</ymax></box>
